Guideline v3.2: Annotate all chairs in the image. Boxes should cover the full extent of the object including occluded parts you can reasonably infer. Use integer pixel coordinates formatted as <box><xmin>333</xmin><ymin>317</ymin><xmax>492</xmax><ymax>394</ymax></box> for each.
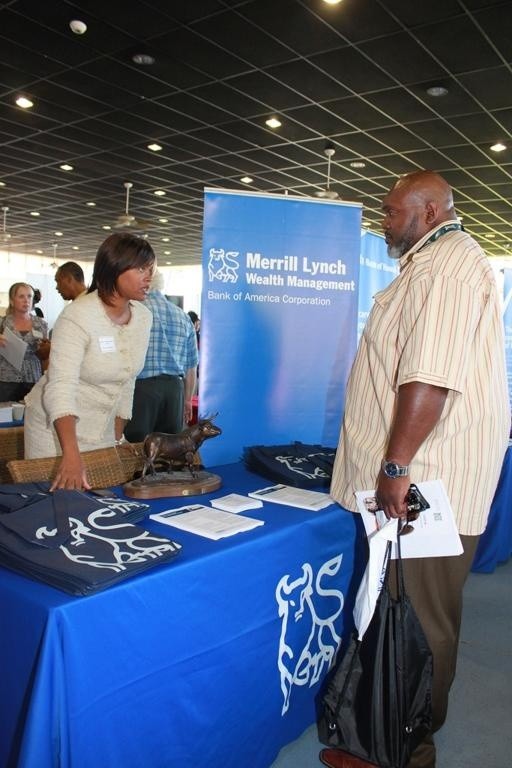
<box><xmin>7</xmin><ymin>442</ymin><xmax>203</xmax><ymax>489</ymax></box>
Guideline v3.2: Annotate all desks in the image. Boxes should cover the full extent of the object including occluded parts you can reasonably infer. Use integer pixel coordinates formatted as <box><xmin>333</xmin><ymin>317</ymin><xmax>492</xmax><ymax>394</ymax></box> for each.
<box><xmin>1</xmin><ymin>461</ymin><xmax>372</xmax><ymax>765</ymax></box>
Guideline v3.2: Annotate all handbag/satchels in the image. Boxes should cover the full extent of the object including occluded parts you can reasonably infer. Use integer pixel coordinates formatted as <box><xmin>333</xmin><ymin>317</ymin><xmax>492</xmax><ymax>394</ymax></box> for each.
<box><xmin>0</xmin><ymin>481</ymin><xmax>181</xmax><ymax>596</ymax></box>
<box><xmin>240</xmin><ymin>441</ymin><xmax>336</xmax><ymax>489</ymax></box>
<box><xmin>315</xmin><ymin>584</ymin><xmax>434</xmax><ymax>768</ymax></box>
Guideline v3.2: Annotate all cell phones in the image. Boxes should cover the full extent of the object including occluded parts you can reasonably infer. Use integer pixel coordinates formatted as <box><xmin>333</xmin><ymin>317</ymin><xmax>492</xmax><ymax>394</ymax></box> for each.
<box><xmin>404</xmin><ymin>484</ymin><xmax>430</xmax><ymax>513</ymax></box>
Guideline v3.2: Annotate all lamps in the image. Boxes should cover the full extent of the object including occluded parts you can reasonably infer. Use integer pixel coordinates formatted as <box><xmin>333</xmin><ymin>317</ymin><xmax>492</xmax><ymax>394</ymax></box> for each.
<box><xmin>50</xmin><ymin>243</ymin><xmax>59</xmax><ymax>268</ymax></box>
<box><xmin>0</xmin><ymin>207</ymin><xmax>11</xmax><ymax>243</ymax></box>
<box><xmin>314</xmin><ymin>148</ymin><xmax>338</xmax><ymax>201</ymax></box>
<box><xmin>118</xmin><ymin>182</ymin><xmax>135</xmax><ymax>226</ymax></box>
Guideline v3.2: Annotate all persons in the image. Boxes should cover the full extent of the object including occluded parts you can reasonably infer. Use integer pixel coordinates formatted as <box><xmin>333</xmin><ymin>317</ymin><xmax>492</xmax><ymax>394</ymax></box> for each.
<box><xmin>188</xmin><ymin>311</ymin><xmax>200</xmax><ymax>342</ymax></box>
<box><xmin>318</xmin><ymin>169</ymin><xmax>512</xmax><ymax>768</ymax></box>
<box><xmin>1</xmin><ymin>282</ymin><xmax>50</xmax><ymax>403</ymax></box>
<box><xmin>123</xmin><ymin>269</ymin><xmax>200</xmax><ymax>443</ymax></box>
<box><xmin>22</xmin><ymin>230</ymin><xmax>159</xmax><ymax>491</ymax></box>
<box><xmin>34</xmin><ymin>261</ymin><xmax>88</xmax><ymax>361</ymax></box>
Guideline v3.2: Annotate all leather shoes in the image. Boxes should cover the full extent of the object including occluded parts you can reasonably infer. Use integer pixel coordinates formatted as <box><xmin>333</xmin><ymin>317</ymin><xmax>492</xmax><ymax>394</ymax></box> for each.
<box><xmin>319</xmin><ymin>748</ymin><xmax>378</xmax><ymax>768</ymax></box>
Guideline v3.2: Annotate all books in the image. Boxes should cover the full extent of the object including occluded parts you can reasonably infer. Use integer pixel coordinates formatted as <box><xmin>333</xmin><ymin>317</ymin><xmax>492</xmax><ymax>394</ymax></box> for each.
<box><xmin>248</xmin><ymin>482</ymin><xmax>336</xmax><ymax>512</ymax></box>
<box><xmin>150</xmin><ymin>503</ymin><xmax>266</xmax><ymax>542</ymax></box>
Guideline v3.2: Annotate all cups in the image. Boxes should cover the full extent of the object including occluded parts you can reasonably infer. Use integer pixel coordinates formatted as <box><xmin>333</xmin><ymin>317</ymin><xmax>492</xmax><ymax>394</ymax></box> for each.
<box><xmin>12</xmin><ymin>404</ymin><xmax>26</xmax><ymax>421</ymax></box>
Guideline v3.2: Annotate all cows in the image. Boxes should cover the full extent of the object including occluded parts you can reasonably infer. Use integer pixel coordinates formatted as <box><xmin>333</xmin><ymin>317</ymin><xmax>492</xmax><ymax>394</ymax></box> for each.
<box><xmin>140</xmin><ymin>410</ymin><xmax>222</xmax><ymax>483</ymax></box>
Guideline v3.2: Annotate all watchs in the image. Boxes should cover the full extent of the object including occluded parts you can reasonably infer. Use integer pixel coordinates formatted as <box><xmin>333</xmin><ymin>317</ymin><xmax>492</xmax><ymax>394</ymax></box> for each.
<box><xmin>380</xmin><ymin>456</ymin><xmax>409</xmax><ymax>479</ymax></box>
